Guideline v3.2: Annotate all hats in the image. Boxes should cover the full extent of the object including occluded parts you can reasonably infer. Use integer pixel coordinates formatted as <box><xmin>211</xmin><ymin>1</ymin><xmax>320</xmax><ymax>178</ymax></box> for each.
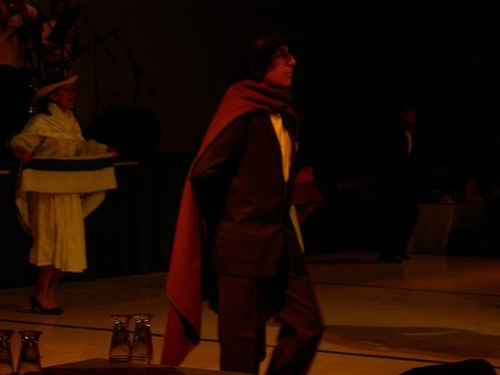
<box><xmin>243</xmin><ymin>30</ymin><xmax>289</xmax><ymax>80</ymax></box>
<box><xmin>36</xmin><ymin>74</ymin><xmax>78</xmax><ymax>97</ymax></box>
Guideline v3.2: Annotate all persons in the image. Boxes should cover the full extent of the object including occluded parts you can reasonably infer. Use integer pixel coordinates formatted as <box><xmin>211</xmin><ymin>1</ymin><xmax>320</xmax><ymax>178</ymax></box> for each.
<box><xmin>376</xmin><ymin>96</ymin><xmax>421</xmax><ymax>264</ymax></box>
<box><xmin>10</xmin><ymin>66</ymin><xmax>119</xmax><ymax>314</ymax></box>
<box><xmin>190</xmin><ymin>24</ymin><xmax>323</xmax><ymax>375</ymax></box>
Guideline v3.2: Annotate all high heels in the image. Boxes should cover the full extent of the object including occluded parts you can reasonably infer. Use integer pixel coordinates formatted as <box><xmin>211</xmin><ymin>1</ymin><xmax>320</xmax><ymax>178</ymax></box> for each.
<box><xmin>29</xmin><ymin>294</ymin><xmax>64</xmax><ymax>315</ymax></box>
